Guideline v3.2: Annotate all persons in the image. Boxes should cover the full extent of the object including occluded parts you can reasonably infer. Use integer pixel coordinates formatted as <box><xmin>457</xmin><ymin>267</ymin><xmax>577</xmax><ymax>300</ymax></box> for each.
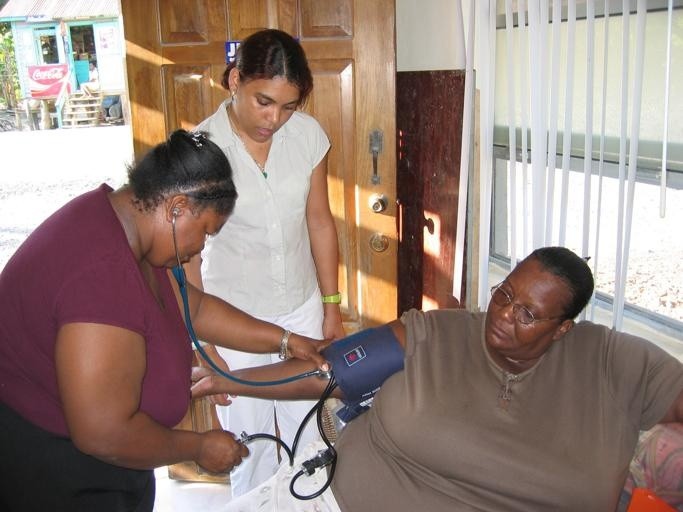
<box><xmin>178</xmin><ymin>27</ymin><xmax>347</xmax><ymax>499</ymax></box>
<box><xmin>0</xmin><ymin>128</ymin><xmax>338</xmax><ymax>511</ymax></box>
<box><xmin>191</xmin><ymin>246</ymin><xmax>682</xmax><ymax>512</ymax></box>
<box><xmin>79</xmin><ymin>63</ymin><xmax>98</xmax><ymax>99</ymax></box>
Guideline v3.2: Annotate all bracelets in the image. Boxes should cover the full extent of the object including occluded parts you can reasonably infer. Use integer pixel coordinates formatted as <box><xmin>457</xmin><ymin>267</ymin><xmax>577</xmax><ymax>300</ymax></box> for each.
<box><xmin>322</xmin><ymin>291</ymin><xmax>342</xmax><ymax>305</ymax></box>
<box><xmin>279</xmin><ymin>330</ymin><xmax>292</xmax><ymax>360</ymax></box>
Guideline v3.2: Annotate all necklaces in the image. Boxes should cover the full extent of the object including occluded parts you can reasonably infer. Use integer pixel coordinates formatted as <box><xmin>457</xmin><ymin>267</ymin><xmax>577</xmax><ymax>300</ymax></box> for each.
<box><xmin>505</xmin><ymin>357</ymin><xmax>523</xmax><ymax>364</ymax></box>
<box><xmin>226</xmin><ymin>104</ymin><xmax>268</xmax><ymax>180</ymax></box>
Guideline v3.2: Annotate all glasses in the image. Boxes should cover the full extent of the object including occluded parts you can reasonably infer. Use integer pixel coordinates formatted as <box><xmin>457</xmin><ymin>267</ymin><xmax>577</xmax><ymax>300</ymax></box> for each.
<box><xmin>489</xmin><ymin>281</ymin><xmax>556</xmax><ymax>326</ymax></box>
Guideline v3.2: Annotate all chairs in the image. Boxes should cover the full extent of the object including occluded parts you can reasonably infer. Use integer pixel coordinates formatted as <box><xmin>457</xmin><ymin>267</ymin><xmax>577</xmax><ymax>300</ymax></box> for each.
<box><xmin>615</xmin><ymin>422</ymin><xmax>683</xmax><ymax>512</ymax></box>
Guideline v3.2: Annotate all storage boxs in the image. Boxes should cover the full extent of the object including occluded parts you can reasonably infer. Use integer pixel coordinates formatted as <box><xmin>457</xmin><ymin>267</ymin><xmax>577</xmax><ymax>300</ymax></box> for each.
<box><xmin>73</xmin><ymin>51</ymin><xmax>88</xmax><ymax>60</ymax></box>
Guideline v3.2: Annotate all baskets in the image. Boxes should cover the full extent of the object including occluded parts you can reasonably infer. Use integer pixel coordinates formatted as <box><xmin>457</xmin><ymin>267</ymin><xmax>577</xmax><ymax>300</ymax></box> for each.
<box><xmin>319</xmin><ymin>399</ymin><xmax>342</xmax><ymax>442</ymax></box>
